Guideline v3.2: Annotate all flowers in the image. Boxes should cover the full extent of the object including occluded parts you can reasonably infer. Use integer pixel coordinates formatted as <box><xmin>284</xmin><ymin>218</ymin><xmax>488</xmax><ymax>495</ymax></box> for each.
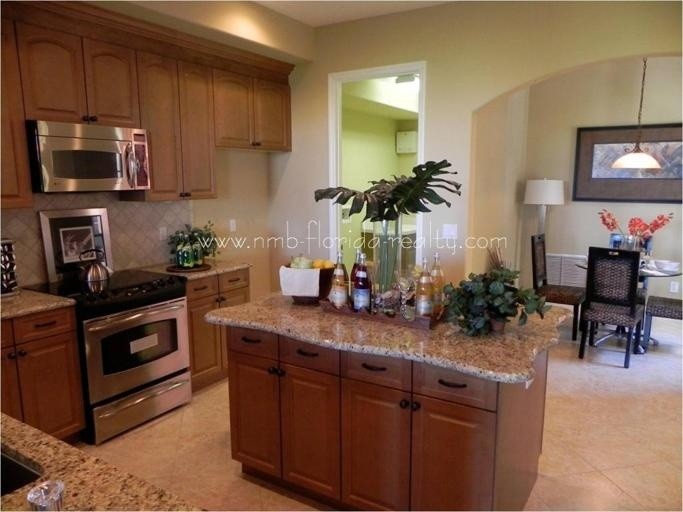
<box><xmin>596</xmin><ymin>208</ymin><xmax>673</xmax><ymax>247</ymax></box>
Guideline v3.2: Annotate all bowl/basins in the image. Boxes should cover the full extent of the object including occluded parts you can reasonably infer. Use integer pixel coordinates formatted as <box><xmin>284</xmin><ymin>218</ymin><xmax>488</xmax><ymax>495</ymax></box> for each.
<box><xmin>283</xmin><ymin>262</ymin><xmax>333</xmax><ymax>303</ymax></box>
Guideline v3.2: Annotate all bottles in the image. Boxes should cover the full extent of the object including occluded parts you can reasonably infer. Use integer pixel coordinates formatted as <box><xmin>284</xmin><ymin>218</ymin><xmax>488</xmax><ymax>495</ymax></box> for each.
<box><xmin>182</xmin><ymin>236</ymin><xmax>194</xmax><ymax>268</ymax></box>
<box><xmin>349</xmin><ymin>249</ymin><xmax>362</xmax><ymax>304</ymax></box>
<box><xmin>193</xmin><ymin>233</ymin><xmax>204</xmax><ymax>267</ymax></box>
<box><xmin>330</xmin><ymin>252</ymin><xmax>349</xmax><ymax>308</ymax></box>
<box><xmin>176</xmin><ymin>232</ymin><xmax>184</xmax><ymax>266</ymax></box>
<box><xmin>353</xmin><ymin>253</ymin><xmax>371</xmax><ymax>315</ymax></box>
<box><xmin>416</xmin><ymin>256</ymin><xmax>432</xmax><ymax>317</ymax></box>
<box><xmin>430</xmin><ymin>252</ymin><xmax>445</xmax><ymax>314</ymax></box>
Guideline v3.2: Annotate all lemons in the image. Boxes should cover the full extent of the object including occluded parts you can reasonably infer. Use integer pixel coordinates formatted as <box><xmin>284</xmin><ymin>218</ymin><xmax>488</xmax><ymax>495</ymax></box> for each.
<box><xmin>313</xmin><ymin>259</ymin><xmax>324</xmax><ymax>269</ymax></box>
<box><xmin>324</xmin><ymin>260</ymin><xmax>334</xmax><ymax>268</ymax></box>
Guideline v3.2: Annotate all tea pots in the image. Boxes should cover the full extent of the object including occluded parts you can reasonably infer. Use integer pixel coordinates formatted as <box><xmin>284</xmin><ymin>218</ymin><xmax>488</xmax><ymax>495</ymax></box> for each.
<box><xmin>72</xmin><ymin>249</ymin><xmax>111</xmax><ymax>282</ymax></box>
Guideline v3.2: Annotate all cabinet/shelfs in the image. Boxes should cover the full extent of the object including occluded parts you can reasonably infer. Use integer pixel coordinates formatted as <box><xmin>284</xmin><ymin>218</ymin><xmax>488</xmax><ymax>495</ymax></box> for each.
<box><xmin>212</xmin><ymin>66</ymin><xmax>292</xmax><ymax>154</ymax></box>
<box><xmin>0</xmin><ymin>17</ymin><xmax>36</xmax><ymax>210</ymax></box>
<box><xmin>185</xmin><ymin>268</ymin><xmax>250</xmax><ymax>393</ymax></box>
<box><xmin>122</xmin><ymin>50</ymin><xmax>217</xmax><ymax>201</ymax></box>
<box><xmin>342</xmin><ymin>348</ymin><xmax>551</xmax><ymax>511</ymax></box>
<box><xmin>361</xmin><ymin>232</ymin><xmax>415</xmax><ymax>285</ymax></box>
<box><xmin>0</xmin><ymin>304</ymin><xmax>85</xmax><ymax>440</ymax></box>
<box><xmin>226</xmin><ymin>327</ymin><xmax>341</xmax><ymax>507</ymax></box>
<box><xmin>15</xmin><ymin>22</ymin><xmax>139</xmax><ymax>128</ymax></box>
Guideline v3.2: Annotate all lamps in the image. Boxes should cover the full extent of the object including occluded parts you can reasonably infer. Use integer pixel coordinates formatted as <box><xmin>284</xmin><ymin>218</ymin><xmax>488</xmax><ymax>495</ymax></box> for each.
<box><xmin>612</xmin><ymin>56</ymin><xmax>661</xmax><ymax>170</ymax></box>
<box><xmin>523</xmin><ymin>178</ymin><xmax>565</xmax><ymax>233</ymax></box>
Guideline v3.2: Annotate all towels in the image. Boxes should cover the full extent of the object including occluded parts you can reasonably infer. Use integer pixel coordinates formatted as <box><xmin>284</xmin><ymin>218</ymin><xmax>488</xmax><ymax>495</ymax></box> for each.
<box><xmin>279</xmin><ymin>265</ymin><xmax>321</xmax><ymax>298</ymax></box>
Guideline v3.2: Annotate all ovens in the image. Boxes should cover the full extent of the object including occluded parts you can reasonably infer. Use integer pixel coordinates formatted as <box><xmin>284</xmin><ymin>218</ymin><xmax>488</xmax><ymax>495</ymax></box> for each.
<box><xmin>76</xmin><ymin>296</ymin><xmax>191</xmax><ymax>445</ymax></box>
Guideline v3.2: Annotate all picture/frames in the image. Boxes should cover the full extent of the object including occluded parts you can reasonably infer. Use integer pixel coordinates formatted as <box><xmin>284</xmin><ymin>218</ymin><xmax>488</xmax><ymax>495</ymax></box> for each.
<box><xmin>39</xmin><ymin>207</ymin><xmax>112</xmax><ymax>284</ymax></box>
<box><xmin>572</xmin><ymin>123</ymin><xmax>683</xmax><ymax>203</ymax></box>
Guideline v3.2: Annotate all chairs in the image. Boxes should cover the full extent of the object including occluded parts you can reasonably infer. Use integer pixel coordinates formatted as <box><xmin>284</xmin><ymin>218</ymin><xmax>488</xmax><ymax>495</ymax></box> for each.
<box><xmin>609</xmin><ymin>232</ymin><xmax>653</xmax><ymax>257</ymax></box>
<box><xmin>642</xmin><ymin>295</ymin><xmax>682</xmax><ymax>350</ymax></box>
<box><xmin>578</xmin><ymin>246</ymin><xmax>644</xmax><ymax>369</ymax></box>
<box><xmin>530</xmin><ymin>233</ymin><xmax>589</xmax><ymax>340</ymax></box>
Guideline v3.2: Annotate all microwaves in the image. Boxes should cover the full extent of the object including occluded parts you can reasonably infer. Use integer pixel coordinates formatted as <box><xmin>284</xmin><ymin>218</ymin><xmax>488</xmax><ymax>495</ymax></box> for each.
<box><xmin>25</xmin><ymin>119</ymin><xmax>151</xmax><ymax>193</ymax></box>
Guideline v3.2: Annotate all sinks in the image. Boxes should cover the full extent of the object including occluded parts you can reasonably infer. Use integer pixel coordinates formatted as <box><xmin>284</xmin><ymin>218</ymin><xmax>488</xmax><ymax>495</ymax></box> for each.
<box><xmin>2</xmin><ymin>443</ymin><xmax>45</xmax><ymax>495</ymax></box>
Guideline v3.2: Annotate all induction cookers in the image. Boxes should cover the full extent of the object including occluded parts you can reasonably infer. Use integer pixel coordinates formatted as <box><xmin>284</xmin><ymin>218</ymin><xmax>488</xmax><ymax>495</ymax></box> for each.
<box><xmin>20</xmin><ymin>270</ymin><xmax>186</xmax><ymax>306</ymax></box>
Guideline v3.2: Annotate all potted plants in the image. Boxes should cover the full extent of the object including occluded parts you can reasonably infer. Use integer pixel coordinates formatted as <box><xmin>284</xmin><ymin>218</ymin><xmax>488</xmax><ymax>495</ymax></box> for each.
<box><xmin>446</xmin><ymin>266</ymin><xmax>550</xmax><ymax>336</ymax></box>
<box><xmin>167</xmin><ymin>221</ymin><xmax>221</xmax><ymax>267</ymax></box>
<box><xmin>314</xmin><ymin>160</ymin><xmax>461</xmax><ymax>298</ymax></box>
